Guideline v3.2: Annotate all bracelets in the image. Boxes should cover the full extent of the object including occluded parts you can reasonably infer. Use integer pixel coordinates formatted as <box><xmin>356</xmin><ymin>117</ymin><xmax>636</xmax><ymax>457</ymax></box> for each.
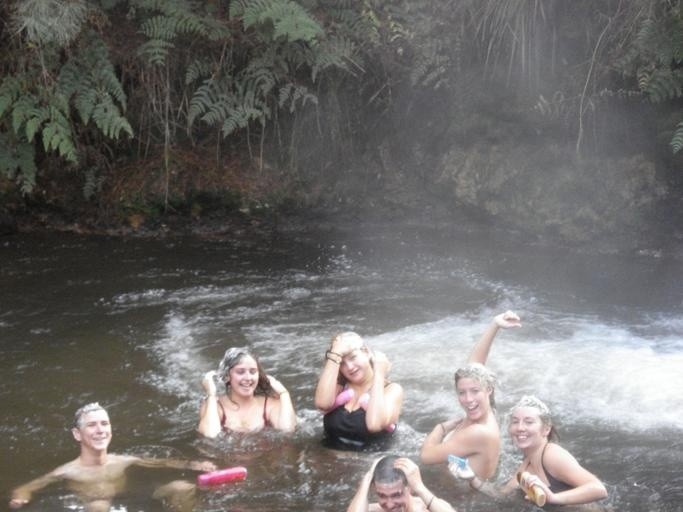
<box><xmin>426</xmin><ymin>494</ymin><xmax>435</xmax><ymax>510</ymax></box>
<box><xmin>277</xmin><ymin>389</ymin><xmax>288</xmax><ymax>395</ymax></box>
<box><xmin>439</xmin><ymin>423</ymin><xmax>446</xmax><ymax>437</ymax></box>
<box><xmin>476</xmin><ymin>480</ymin><xmax>485</xmax><ymax>490</ymax></box>
<box><xmin>324</xmin><ymin>350</ymin><xmax>342</xmax><ymax>365</ymax></box>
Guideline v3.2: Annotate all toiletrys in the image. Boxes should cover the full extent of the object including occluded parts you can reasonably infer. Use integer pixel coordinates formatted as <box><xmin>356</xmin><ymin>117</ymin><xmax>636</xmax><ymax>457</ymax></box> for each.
<box><xmin>357</xmin><ymin>393</ymin><xmax>396</xmax><ymax>434</ymax></box>
<box><xmin>517</xmin><ymin>471</ymin><xmax>547</xmax><ymax>509</ymax></box>
<box><xmin>197</xmin><ymin>465</ymin><xmax>248</xmax><ymax>486</ymax></box>
<box><xmin>445</xmin><ymin>453</ymin><xmax>469</xmax><ymax>472</ymax></box>
<box><xmin>319</xmin><ymin>388</ymin><xmax>354</xmax><ymax>414</ymax></box>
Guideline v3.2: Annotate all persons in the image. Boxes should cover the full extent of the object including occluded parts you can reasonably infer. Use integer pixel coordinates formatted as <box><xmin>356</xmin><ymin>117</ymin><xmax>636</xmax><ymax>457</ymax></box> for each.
<box><xmin>5</xmin><ymin>399</ymin><xmax>213</xmax><ymax>510</ymax></box>
<box><xmin>189</xmin><ymin>347</ymin><xmax>298</xmax><ymax>434</ymax></box>
<box><xmin>419</xmin><ymin>307</ymin><xmax>522</xmax><ymax>484</ymax></box>
<box><xmin>343</xmin><ymin>453</ymin><xmax>457</xmax><ymax>511</ymax></box>
<box><xmin>445</xmin><ymin>394</ymin><xmax>611</xmax><ymax>511</ymax></box>
<box><xmin>313</xmin><ymin>331</ymin><xmax>402</xmax><ymax>452</ymax></box>
